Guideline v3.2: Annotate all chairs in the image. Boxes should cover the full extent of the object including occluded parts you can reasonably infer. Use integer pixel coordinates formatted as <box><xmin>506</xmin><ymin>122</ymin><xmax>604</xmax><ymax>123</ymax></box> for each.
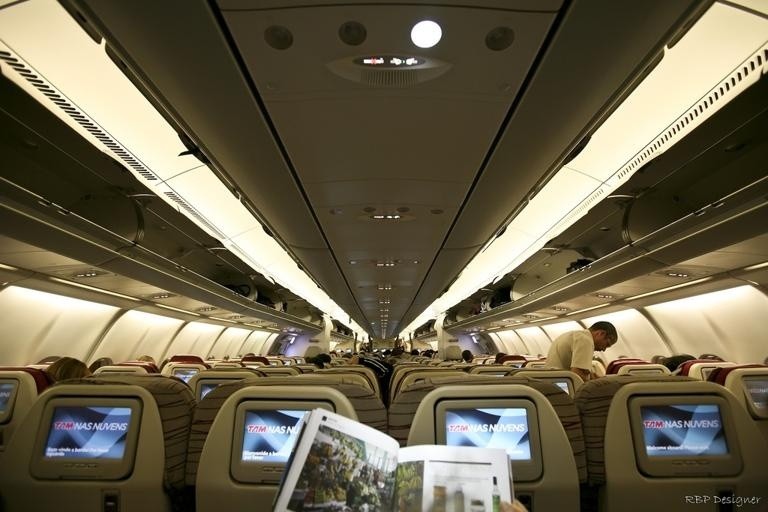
<box><xmin>0</xmin><ymin>354</ymin><xmax>768</xmax><ymax>511</ymax></box>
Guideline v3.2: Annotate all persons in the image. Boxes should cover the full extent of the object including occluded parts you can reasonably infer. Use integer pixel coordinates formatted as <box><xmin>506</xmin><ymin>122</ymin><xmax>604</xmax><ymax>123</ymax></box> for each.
<box><xmin>460</xmin><ymin>349</ymin><xmax>474</xmax><ymax>362</ymax></box>
<box><xmin>304</xmin><ymin>331</ymin><xmax>437</xmax><ymax>370</ymax></box>
<box><xmin>544</xmin><ymin>320</ymin><xmax>618</xmax><ymax>380</ymax></box>
<box><xmin>500</xmin><ymin>498</ymin><xmax>529</xmax><ymax>511</ymax></box>
<box><xmin>349</xmin><ymin>356</ymin><xmax>394</xmax><ymax>408</ymax></box>
<box><xmin>46</xmin><ymin>357</ymin><xmax>91</xmax><ymax>382</ymax></box>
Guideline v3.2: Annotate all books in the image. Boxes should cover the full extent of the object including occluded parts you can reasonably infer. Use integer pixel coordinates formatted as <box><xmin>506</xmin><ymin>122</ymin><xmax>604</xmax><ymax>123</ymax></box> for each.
<box><xmin>270</xmin><ymin>405</ymin><xmax>516</xmax><ymax>512</ymax></box>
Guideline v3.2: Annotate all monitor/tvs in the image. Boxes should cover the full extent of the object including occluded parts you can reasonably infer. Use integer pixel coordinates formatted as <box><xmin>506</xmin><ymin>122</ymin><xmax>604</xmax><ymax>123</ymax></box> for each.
<box><xmin>629</xmin><ymin>394</ymin><xmax>743</xmax><ymax>478</ymax></box>
<box><xmin>0</xmin><ymin>379</ymin><xmax>19</xmax><ymax>423</ymax></box>
<box><xmin>434</xmin><ymin>398</ymin><xmax>544</xmax><ymax>483</ymax></box>
<box><xmin>229</xmin><ymin>398</ymin><xmax>336</xmax><ymax>484</ymax></box>
<box><xmin>171</xmin><ymin>353</ymin><xmax>716</xmax><ymax>405</ymax></box>
<box><xmin>742</xmin><ymin>376</ymin><xmax>768</xmax><ymax>421</ymax></box>
<box><xmin>29</xmin><ymin>396</ymin><xmax>143</xmax><ymax>480</ymax></box>
